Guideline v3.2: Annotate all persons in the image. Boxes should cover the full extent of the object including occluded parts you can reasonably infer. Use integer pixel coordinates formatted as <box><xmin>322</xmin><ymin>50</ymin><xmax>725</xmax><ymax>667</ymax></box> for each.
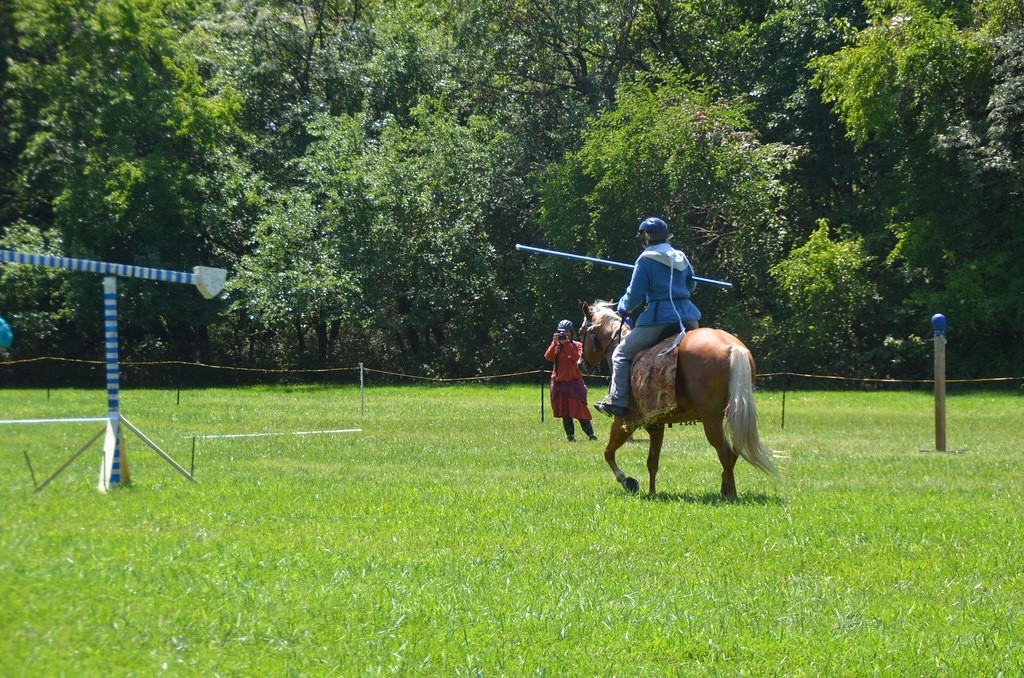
<box><xmin>544</xmin><ymin>319</ymin><xmax>598</xmax><ymax>443</ymax></box>
<box><xmin>593</xmin><ymin>217</ymin><xmax>701</xmax><ymax>417</ymax></box>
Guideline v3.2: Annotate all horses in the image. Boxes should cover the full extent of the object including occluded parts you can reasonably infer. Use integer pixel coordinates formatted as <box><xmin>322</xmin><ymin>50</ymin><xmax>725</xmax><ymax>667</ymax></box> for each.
<box><xmin>576</xmin><ymin>298</ymin><xmax>790</xmax><ymax>503</ymax></box>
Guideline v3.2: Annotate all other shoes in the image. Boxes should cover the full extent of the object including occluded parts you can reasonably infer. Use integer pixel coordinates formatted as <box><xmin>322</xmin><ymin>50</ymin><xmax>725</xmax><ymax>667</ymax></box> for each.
<box><xmin>589</xmin><ymin>436</ymin><xmax>598</xmax><ymax>441</ymax></box>
<box><xmin>594</xmin><ymin>400</ymin><xmax>627</xmax><ymax>418</ymax></box>
<box><xmin>567</xmin><ymin>436</ymin><xmax>576</xmax><ymax>443</ymax></box>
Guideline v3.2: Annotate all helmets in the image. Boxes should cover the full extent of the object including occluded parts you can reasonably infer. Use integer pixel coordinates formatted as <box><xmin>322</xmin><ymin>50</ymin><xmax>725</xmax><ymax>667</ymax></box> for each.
<box><xmin>636</xmin><ymin>217</ymin><xmax>669</xmax><ymax>241</ymax></box>
<box><xmin>558</xmin><ymin>320</ymin><xmax>574</xmax><ymax>337</ymax></box>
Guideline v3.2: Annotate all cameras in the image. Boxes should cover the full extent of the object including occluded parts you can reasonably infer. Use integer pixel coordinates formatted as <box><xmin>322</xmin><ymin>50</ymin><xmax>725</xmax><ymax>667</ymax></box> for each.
<box><xmin>557</xmin><ymin>333</ymin><xmax>566</xmax><ymax>340</ymax></box>
